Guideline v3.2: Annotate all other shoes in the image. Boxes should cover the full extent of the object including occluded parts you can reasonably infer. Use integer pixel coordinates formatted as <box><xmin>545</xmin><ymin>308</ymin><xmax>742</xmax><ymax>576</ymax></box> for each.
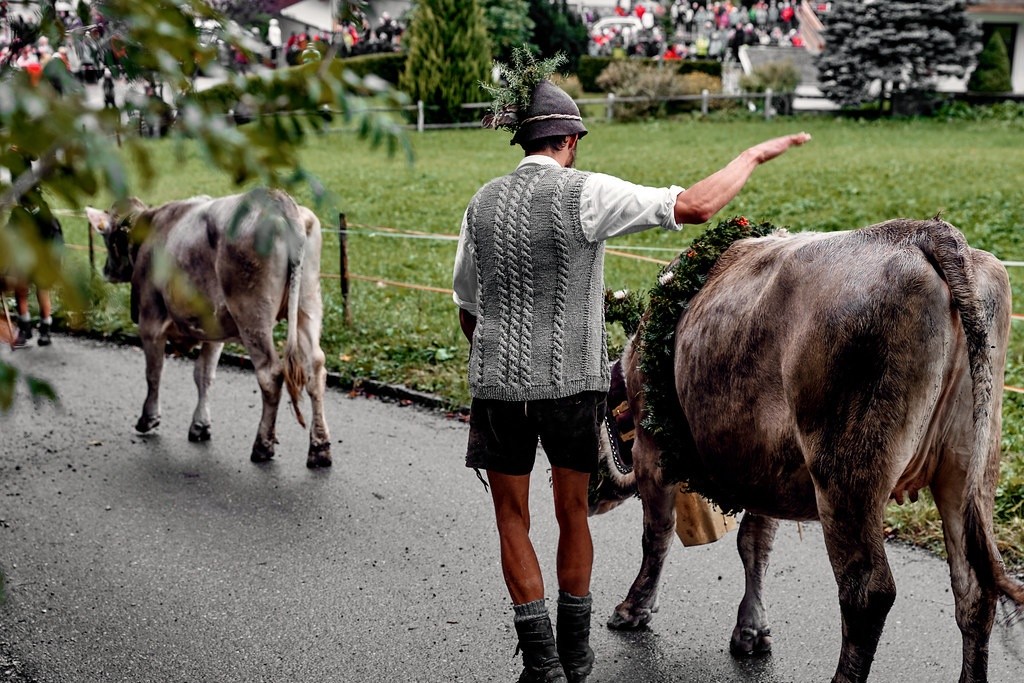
<box><xmin>14</xmin><ymin>329</ymin><xmax>32</xmax><ymax>346</ymax></box>
<box><xmin>37</xmin><ymin>331</ymin><xmax>50</xmax><ymax>345</ymax></box>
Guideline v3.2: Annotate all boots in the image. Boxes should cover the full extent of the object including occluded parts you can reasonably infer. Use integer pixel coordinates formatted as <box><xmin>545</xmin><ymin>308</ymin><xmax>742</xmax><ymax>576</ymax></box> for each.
<box><xmin>512</xmin><ymin>598</ymin><xmax>565</xmax><ymax>683</ymax></box>
<box><xmin>553</xmin><ymin>590</ymin><xmax>595</xmax><ymax>680</ymax></box>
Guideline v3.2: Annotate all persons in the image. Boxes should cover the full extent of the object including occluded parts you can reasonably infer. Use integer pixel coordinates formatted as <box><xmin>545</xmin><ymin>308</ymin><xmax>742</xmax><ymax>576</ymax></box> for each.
<box><xmin>0</xmin><ymin>120</ymin><xmax>40</xmax><ymax>205</ymax></box>
<box><xmin>450</xmin><ymin>39</ymin><xmax>812</xmax><ymax>683</ymax></box>
<box><xmin>0</xmin><ymin>183</ymin><xmax>64</xmax><ymax>350</ymax></box>
<box><xmin>1</xmin><ymin>0</ymin><xmax>803</xmax><ymax>109</ymax></box>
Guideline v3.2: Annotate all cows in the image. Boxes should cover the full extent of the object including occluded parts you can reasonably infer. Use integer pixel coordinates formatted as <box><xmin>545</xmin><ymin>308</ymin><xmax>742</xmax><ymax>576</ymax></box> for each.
<box><xmin>84</xmin><ymin>186</ymin><xmax>334</xmax><ymax>471</ymax></box>
<box><xmin>584</xmin><ymin>216</ymin><xmax>1024</xmax><ymax>683</ymax></box>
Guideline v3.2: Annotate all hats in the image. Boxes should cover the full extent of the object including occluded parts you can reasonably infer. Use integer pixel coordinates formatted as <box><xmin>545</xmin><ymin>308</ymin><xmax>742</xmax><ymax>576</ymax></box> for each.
<box><xmin>510</xmin><ymin>78</ymin><xmax>587</xmax><ymax>146</ymax></box>
